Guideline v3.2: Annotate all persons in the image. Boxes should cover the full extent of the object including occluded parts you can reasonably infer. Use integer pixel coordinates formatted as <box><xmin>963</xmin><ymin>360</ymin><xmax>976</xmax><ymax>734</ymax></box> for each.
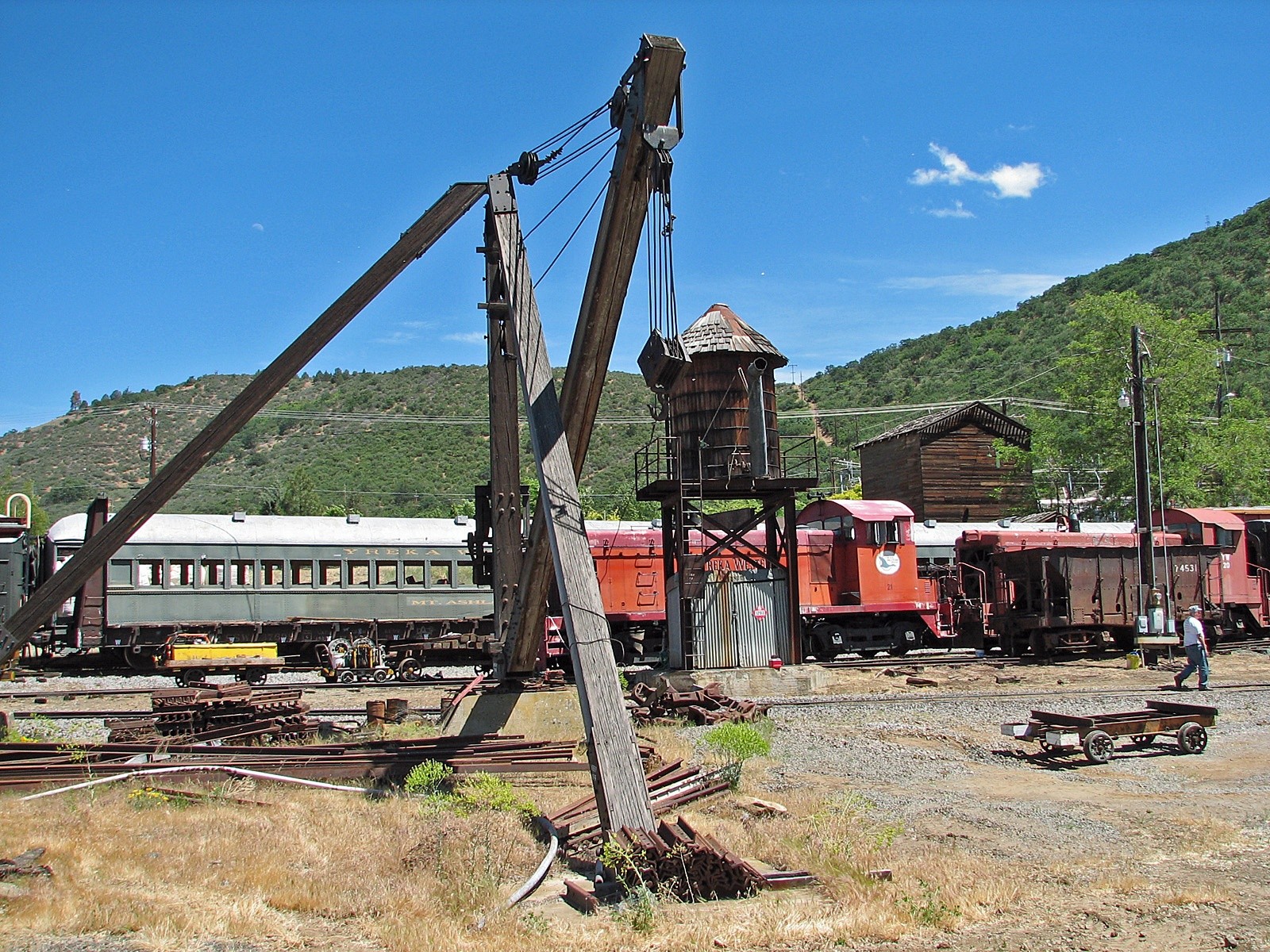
<box><xmin>1174</xmin><ymin>606</ymin><xmax>1215</xmax><ymax>691</ymax></box>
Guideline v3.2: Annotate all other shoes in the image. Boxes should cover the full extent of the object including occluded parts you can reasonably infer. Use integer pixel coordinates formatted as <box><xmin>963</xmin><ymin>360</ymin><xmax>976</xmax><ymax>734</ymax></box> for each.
<box><xmin>1199</xmin><ymin>685</ymin><xmax>1213</xmax><ymax>691</ymax></box>
<box><xmin>1174</xmin><ymin>676</ymin><xmax>1182</xmax><ymax>689</ymax></box>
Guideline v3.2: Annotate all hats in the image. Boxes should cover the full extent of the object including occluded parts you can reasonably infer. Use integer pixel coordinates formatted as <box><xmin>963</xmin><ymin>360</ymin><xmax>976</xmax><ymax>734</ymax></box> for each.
<box><xmin>1188</xmin><ymin>605</ymin><xmax>1202</xmax><ymax>612</ymax></box>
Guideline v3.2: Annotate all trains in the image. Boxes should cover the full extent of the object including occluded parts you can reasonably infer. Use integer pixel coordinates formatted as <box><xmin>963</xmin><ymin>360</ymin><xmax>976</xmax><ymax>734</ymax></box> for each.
<box><xmin>0</xmin><ymin>492</ymin><xmax>1270</xmax><ymax>687</ymax></box>
